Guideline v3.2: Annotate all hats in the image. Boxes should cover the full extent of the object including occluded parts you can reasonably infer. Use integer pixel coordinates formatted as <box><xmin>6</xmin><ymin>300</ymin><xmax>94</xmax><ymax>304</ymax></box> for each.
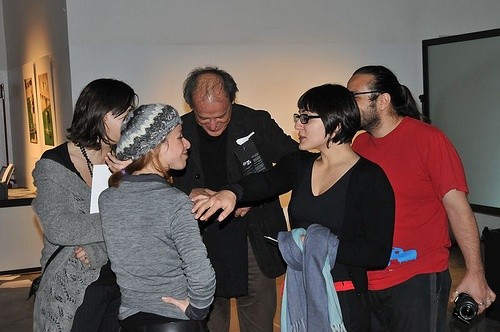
<box><xmin>115</xmin><ymin>103</ymin><xmax>182</xmax><ymax>161</ymax></box>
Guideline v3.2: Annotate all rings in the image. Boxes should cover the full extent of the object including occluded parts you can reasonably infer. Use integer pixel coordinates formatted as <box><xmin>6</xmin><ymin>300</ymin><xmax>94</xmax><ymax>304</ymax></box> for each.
<box><xmin>486</xmin><ymin>298</ymin><xmax>491</xmax><ymax>303</ymax></box>
<box><xmin>84</xmin><ymin>255</ymin><xmax>87</xmax><ymax>260</ymax></box>
<box><xmin>477</xmin><ymin>301</ymin><xmax>484</xmax><ymax>306</ymax></box>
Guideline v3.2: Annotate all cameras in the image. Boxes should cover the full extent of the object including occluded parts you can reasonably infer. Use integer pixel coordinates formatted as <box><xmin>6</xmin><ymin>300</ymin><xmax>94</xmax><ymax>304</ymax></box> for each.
<box><xmin>452</xmin><ymin>293</ymin><xmax>479</xmax><ymax>326</ymax></box>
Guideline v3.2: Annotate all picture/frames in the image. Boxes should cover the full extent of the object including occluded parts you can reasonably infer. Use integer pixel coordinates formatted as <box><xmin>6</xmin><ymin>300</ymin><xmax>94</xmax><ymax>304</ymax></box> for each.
<box><xmin>20</xmin><ymin>54</ymin><xmax>61</xmax><ymax>161</ymax></box>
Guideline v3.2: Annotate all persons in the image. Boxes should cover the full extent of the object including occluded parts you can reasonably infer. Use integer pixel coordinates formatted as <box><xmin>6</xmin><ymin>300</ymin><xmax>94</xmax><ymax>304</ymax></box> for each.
<box><xmin>348</xmin><ymin>66</ymin><xmax>496</xmax><ymax>332</ymax></box>
<box><xmin>33</xmin><ymin>78</ymin><xmax>139</xmax><ymax>332</ymax></box>
<box><xmin>168</xmin><ymin>65</ymin><xmax>312</xmax><ymax>332</ymax></box>
<box><xmin>98</xmin><ymin>103</ymin><xmax>216</xmax><ymax>332</ymax></box>
<box><xmin>191</xmin><ymin>82</ymin><xmax>396</xmax><ymax>332</ymax></box>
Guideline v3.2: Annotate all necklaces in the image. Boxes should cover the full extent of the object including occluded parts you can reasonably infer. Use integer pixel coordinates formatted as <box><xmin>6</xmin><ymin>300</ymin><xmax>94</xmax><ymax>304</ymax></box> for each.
<box><xmin>79</xmin><ymin>145</ymin><xmax>113</xmax><ymax>178</ymax></box>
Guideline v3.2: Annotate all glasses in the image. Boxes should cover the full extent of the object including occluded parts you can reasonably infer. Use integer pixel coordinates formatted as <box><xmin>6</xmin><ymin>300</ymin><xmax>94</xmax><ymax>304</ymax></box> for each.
<box><xmin>293</xmin><ymin>113</ymin><xmax>322</xmax><ymax>125</ymax></box>
